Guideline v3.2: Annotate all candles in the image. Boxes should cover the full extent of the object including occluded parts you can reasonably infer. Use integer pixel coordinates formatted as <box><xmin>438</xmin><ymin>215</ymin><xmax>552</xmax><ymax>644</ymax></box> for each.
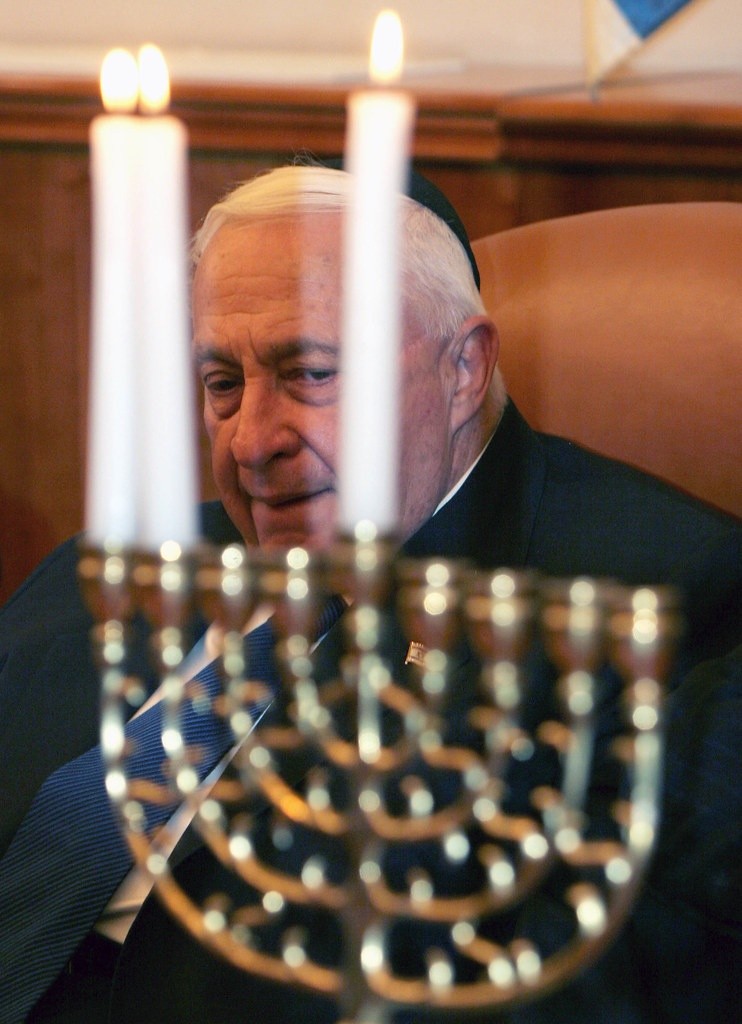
<box><xmin>78</xmin><ymin>46</ymin><xmax>197</xmax><ymax>545</ymax></box>
<box><xmin>332</xmin><ymin>13</ymin><xmax>413</xmax><ymax>534</ymax></box>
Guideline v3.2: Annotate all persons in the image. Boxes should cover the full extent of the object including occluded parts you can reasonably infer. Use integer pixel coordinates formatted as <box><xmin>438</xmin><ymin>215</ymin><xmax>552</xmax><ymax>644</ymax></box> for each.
<box><xmin>0</xmin><ymin>150</ymin><xmax>742</xmax><ymax>1024</ymax></box>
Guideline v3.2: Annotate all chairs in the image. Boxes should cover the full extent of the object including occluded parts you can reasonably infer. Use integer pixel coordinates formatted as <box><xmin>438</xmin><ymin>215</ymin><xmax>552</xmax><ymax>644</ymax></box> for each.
<box><xmin>468</xmin><ymin>200</ymin><xmax>742</xmax><ymax>531</ymax></box>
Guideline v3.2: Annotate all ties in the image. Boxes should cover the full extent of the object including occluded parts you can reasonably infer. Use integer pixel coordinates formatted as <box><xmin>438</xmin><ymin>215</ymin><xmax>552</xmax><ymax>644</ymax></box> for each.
<box><xmin>1</xmin><ymin>590</ymin><xmax>349</xmax><ymax>1024</ymax></box>
